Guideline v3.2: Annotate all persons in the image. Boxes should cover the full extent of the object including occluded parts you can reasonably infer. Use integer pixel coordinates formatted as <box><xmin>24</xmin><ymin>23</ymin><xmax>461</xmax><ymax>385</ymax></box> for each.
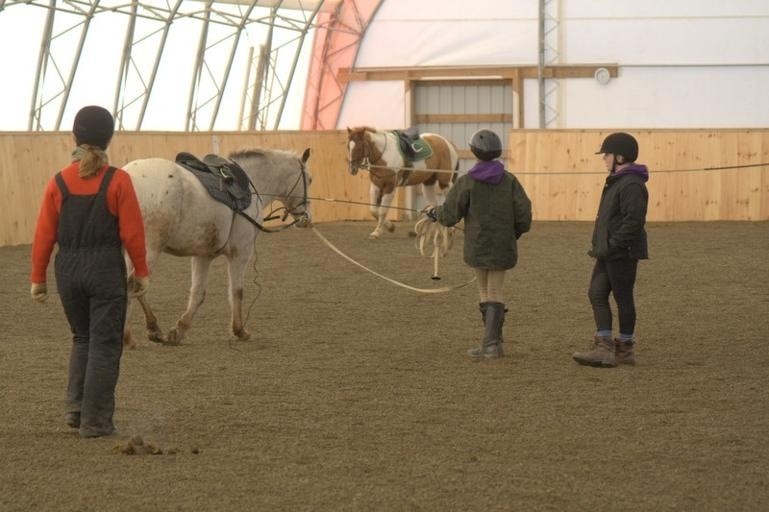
<box><xmin>573</xmin><ymin>132</ymin><xmax>648</xmax><ymax>367</ymax></box>
<box><xmin>30</xmin><ymin>106</ymin><xmax>149</xmax><ymax>439</ymax></box>
<box><xmin>427</xmin><ymin>129</ymin><xmax>532</xmax><ymax>358</ymax></box>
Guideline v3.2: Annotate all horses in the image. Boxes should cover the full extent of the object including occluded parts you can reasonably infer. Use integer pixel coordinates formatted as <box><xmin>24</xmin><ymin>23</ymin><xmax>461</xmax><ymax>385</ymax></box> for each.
<box><xmin>344</xmin><ymin>125</ymin><xmax>461</xmax><ymax>241</ymax></box>
<box><xmin>117</xmin><ymin>147</ymin><xmax>314</xmax><ymax>350</ymax></box>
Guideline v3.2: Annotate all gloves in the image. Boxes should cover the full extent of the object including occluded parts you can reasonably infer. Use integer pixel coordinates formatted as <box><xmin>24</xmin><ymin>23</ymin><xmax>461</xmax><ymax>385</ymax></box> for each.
<box><xmin>128</xmin><ymin>267</ymin><xmax>149</xmax><ymax>298</ymax></box>
<box><xmin>31</xmin><ymin>282</ymin><xmax>49</xmax><ymax>303</ymax></box>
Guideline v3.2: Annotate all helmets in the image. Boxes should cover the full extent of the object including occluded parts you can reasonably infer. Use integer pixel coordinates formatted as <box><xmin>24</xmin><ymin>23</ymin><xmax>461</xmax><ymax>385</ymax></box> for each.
<box><xmin>594</xmin><ymin>133</ymin><xmax>638</xmax><ymax>162</ymax></box>
<box><xmin>73</xmin><ymin>106</ymin><xmax>114</xmax><ymax>150</ymax></box>
<box><xmin>469</xmin><ymin>129</ymin><xmax>502</xmax><ymax>160</ymax></box>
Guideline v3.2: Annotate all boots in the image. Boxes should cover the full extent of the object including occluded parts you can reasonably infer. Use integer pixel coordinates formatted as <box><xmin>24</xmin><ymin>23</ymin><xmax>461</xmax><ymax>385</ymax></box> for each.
<box><xmin>468</xmin><ymin>302</ymin><xmax>508</xmax><ymax>359</ymax></box>
<box><xmin>614</xmin><ymin>337</ymin><xmax>636</xmax><ymax>365</ymax></box>
<box><xmin>573</xmin><ymin>336</ymin><xmax>617</xmax><ymax>368</ymax></box>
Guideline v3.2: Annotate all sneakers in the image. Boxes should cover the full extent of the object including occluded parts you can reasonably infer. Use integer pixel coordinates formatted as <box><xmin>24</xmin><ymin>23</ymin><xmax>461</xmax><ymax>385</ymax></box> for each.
<box><xmin>64</xmin><ymin>413</ymin><xmax>119</xmax><ymax>438</ymax></box>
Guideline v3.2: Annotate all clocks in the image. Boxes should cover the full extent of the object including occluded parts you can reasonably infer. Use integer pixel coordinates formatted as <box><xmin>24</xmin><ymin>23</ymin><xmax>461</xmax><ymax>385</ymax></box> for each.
<box><xmin>595</xmin><ymin>68</ymin><xmax>611</xmax><ymax>85</ymax></box>
